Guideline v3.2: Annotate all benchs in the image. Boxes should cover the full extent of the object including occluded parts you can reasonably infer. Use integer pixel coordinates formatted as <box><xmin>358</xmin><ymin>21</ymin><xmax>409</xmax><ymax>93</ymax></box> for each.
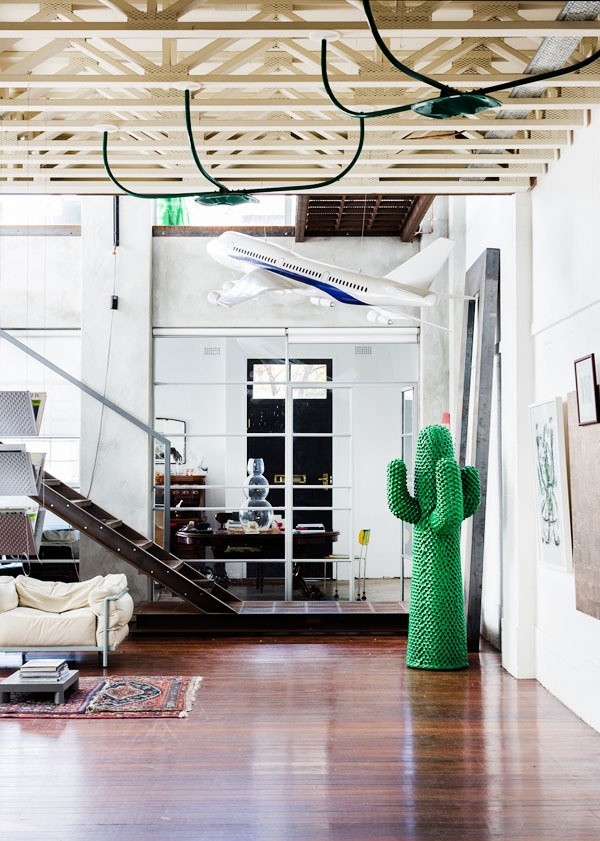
<box><xmin>0</xmin><ymin>575</ymin><xmax>134</xmax><ymax>670</ymax></box>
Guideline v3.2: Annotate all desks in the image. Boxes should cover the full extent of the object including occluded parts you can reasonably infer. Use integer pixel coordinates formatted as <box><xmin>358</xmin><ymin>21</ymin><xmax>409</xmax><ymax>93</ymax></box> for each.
<box><xmin>0</xmin><ymin>670</ymin><xmax>80</xmax><ymax>705</ymax></box>
<box><xmin>176</xmin><ymin>531</ymin><xmax>338</xmax><ymax>593</ymax></box>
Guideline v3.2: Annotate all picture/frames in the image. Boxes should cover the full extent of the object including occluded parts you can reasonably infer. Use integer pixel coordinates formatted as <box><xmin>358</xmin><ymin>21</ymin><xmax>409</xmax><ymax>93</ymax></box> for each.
<box><xmin>574</xmin><ymin>353</ymin><xmax>600</xmax><ymax>426</ymax></box>
<box><xmin>528</xmin><ymin>398</ymin><xmax>573</xmax><ymax>572</ymax></box>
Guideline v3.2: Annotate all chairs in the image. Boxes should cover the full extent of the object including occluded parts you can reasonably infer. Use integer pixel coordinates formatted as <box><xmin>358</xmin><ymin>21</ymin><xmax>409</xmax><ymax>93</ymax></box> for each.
<box><xmin>324</xmin><ymin>530</ymin><xmax>370</xmax><ymax>601</ymax></box>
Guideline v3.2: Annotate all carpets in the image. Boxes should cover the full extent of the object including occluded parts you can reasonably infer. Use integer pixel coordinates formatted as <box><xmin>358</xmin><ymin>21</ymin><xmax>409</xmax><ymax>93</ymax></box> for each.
<box><xmin>0</xmin><ymin>676</ymin><xmax>204</xmax><ymax>718</ymax></box>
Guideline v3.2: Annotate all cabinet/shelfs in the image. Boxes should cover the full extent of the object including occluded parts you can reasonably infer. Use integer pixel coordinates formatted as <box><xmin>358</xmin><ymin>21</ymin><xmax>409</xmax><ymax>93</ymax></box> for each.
<box><xmin>155</xmin><ymin>476</ymin><xmax>206</xmax><ymax>547</ymax></box>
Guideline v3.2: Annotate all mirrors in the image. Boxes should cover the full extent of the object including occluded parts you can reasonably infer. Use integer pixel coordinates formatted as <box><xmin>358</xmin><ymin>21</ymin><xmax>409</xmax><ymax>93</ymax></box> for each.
<box><xmin>155</xmin><ymin>417</ymin><xmax>186</xmax><ymax>464</ymax></box>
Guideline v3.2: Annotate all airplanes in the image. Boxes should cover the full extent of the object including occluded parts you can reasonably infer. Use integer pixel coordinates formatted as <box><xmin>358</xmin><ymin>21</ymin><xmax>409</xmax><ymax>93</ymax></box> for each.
<box><xmin>206</xmin><ymin>230</ymin><xmax>455</xmax><ymax>331</ymax></box>
